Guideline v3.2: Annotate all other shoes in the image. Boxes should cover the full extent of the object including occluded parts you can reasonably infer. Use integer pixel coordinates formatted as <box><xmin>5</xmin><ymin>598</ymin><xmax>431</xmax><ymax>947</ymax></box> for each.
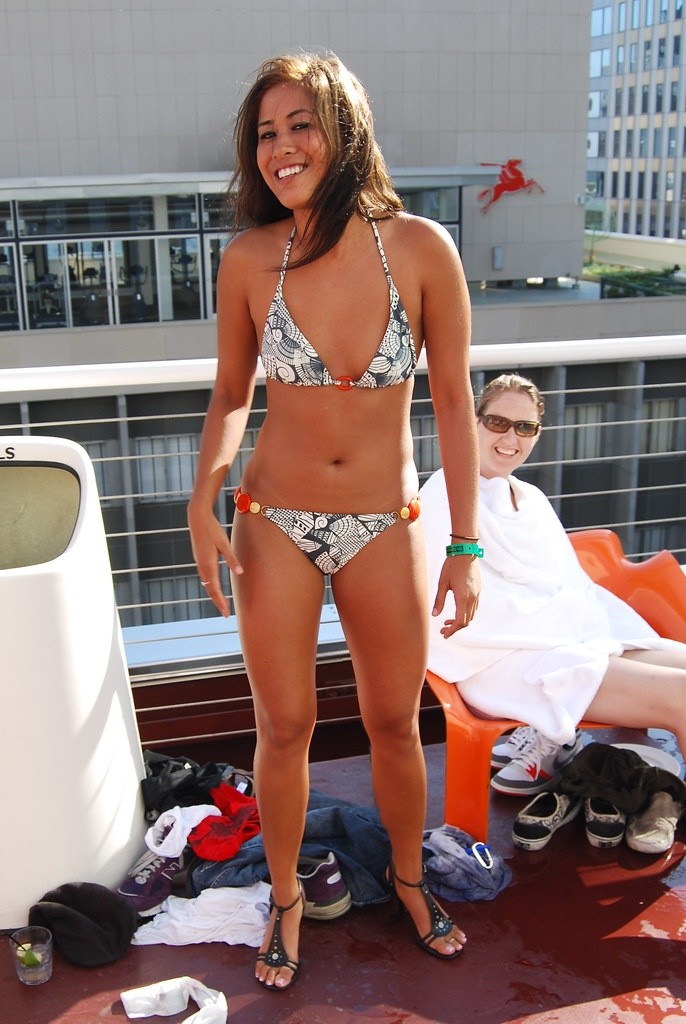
<box><xmin>625</xmin><ymin>791</ymin><xmax>685</xmax><ymax>854</ymax></box>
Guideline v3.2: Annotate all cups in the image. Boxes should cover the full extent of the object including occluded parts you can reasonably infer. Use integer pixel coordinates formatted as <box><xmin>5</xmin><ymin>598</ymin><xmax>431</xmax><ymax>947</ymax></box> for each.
<box><xmin>9</xmin><ymin>926</ymin><xmax>53</xmax><ymax>986</ymax></box>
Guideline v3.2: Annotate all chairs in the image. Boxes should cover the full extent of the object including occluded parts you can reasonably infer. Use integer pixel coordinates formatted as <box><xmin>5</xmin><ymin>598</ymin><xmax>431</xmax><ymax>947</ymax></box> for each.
<box><xmin>424</xmin><ymin>528</ymin><xmax>685</xmax><ymax>840</ymax></box>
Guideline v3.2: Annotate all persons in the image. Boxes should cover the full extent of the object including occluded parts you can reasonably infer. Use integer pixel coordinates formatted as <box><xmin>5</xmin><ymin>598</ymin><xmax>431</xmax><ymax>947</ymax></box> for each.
<box><xmin>187</xmin><ymin>53</ymin><xmax>483</xmax><ymax>991</ymax></box>
<box><xmin>419</xmin><ymin>374</ymin><xmax>686</xmax><ymax>761</ymax></box>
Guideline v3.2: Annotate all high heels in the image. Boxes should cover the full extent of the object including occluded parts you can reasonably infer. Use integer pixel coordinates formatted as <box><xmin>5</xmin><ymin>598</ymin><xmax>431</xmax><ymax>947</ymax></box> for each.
<box><xmin>384</xmin><ymin>858</ymin><xmax>465</xmax><ymax>961</ymax></box>
<box><xmin>254</xmin><ymin>876</ymin><xmax>306</xmax><ymax>991</ymax></box>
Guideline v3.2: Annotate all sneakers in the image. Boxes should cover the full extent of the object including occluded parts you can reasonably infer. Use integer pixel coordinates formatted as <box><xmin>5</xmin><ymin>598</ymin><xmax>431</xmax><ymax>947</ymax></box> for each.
<box><xmin>511</xmin><ymin>790</ymin><xmax>584</xmax><ymax>851</ymax></box>
<box><xmin>584</xmin><ymin>797</ymin><xmax>626</xmax><ymax>848</ymax></box>
<box><xmin>490</xmin><ymin>726</ymin><xmax>537</xmax><ymax>770</ymax></box>
<box><xmin>111</xmin><ymin>825</ymin><xmax>204</xmax><ymax>917</ymax></box>
<box><xmin>262</xmin><ymin>852</ymin><xmax>352</xmax><ymax>921</ymax></box>
<box><xmin>490</xmin><ymin>727</ymin><xmax>584</xmax><ymax>797</ymax></box>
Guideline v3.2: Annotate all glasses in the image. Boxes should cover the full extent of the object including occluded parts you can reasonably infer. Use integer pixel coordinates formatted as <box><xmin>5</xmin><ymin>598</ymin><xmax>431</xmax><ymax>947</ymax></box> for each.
<box><xmin>478</xmin><ymin>412</ymin><xmax>542</xmax><ymax>437</ymax></box>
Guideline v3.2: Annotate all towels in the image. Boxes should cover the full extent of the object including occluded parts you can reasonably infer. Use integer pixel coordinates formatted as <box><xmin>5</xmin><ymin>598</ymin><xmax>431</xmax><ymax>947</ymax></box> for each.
<box><xmin>415</xmin><ymin>467</ymin><xmax>670</xmax><ymax>746</ymax></box>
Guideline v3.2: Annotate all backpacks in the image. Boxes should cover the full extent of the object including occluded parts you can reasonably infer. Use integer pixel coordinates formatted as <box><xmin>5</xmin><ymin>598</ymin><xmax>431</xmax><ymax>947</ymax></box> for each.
<box><xmin>140</xmin><ymin>749</ymin><xmax>256</xmax><ymax>827</ymax></box>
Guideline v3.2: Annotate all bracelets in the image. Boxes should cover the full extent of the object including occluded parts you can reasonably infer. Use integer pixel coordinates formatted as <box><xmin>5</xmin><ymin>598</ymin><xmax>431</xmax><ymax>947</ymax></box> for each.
<box><xmin>450</xmin><ymin>534</ymin><xmax>480</xmax><ymax>540</ymax></box>
<box><xmin>446</xmin><ymin>543</ymin><xmax>483</xmax><ymax>558</ymax></box>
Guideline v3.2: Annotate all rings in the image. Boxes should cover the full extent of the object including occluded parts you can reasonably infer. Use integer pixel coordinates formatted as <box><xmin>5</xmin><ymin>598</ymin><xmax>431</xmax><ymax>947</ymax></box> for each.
<box><xmin>201</xmin><ymin>582</ymin><xmax>209</xmax><ymax>587</ymax></box>
